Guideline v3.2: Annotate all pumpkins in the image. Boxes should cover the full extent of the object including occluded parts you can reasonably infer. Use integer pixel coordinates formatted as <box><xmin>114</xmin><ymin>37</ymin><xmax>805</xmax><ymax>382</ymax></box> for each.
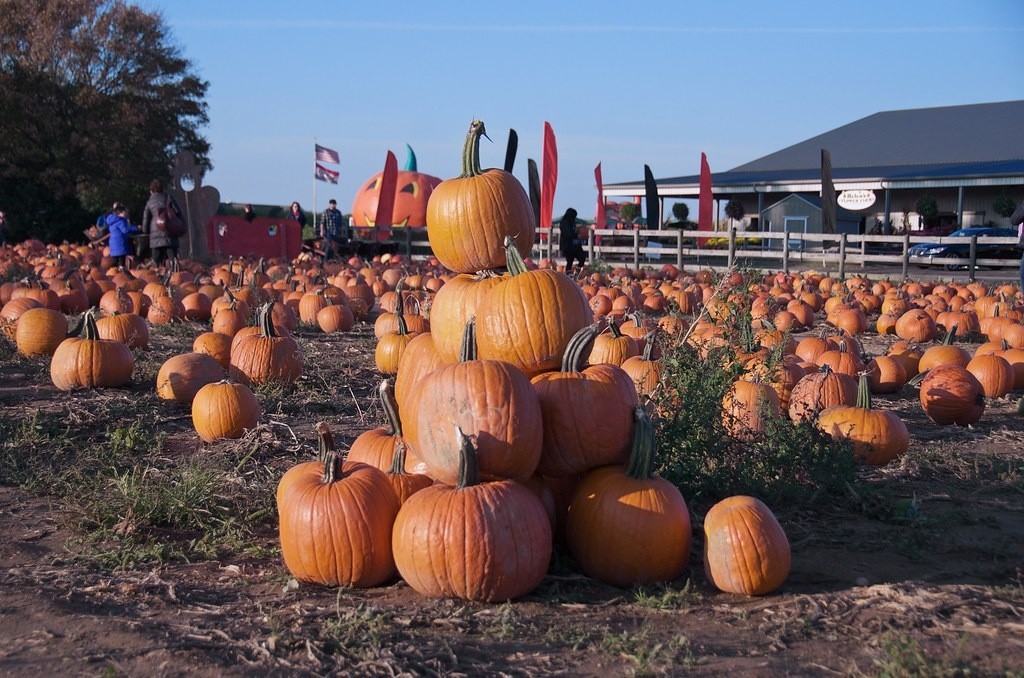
<box><xmin>522</xmin><ymin>258</ymin><xmax>1024</xmax><ymax>467</ymax></box>
<box><xmin>430</xmin><ymin>269</ymin><xmax>512</xmax><ymax>363</ymax></box>
<box><xmin>0</xmin><ymin>240</ymin><xmax>454</xmax><ymax>443</ymax></box>
<box><xmin>475</xmin><ymin>235</ymin><xmax>593</xmax><ymax>372</ymax></box>
<box><xmin>426</xmin><ymin>121</ymin><xmax>535</xmax><ymax>274</ymax></box>
<box><xmin>703</xmin><ymin>496</ymin><xmax>791</xmax><ymax>597</ymax></box>
<box><xmin>277</xmin><ymin>316</ymin><xmax>691</xmax><ymax>601</ymax></box>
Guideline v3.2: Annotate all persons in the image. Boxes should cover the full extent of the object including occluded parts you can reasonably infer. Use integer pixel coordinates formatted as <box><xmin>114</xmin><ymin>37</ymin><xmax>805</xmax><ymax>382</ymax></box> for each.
<box><xmin>1010</xmin><ymin>202</ymin><xmax>1024</xmax><ymax>300</ymax></box>
<box><xmin>242</xmin><ymin>204</ymin><xmax>258</xmax><ymax>224</ymax></box>
<box><xmin>0</xmin><ymin>210</ymin><xmax>9</xmax><ymax>246</ymax></box>
<box><xmin>143</xmin><ymin>179</ymin><xmax>181</xmax><ymax>267</ymax></box>
<box><xmin>96</xmin><ymin>201</ymin><xmax>142</xmax><ymax>272</ymax></box>
<box><xmin>320</xmin><ymin>200</ymin><xmax>344</xmax><ymax>267</ymax></box>
<box><xmin>285</xmin><ymin>202</ymin><xmax>307</xmax><ymax>230</ymax></box>
<box><xmin>559</xmin><ymin>207</ymin><xmax>597</xmax><ymax>282</ymax></box>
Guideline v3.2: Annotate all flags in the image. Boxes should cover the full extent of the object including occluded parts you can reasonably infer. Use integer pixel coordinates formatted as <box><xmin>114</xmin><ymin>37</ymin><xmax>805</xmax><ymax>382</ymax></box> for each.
<box><xmin>317</xmin><ymin>144</ymin><xmax>341</xmax><ymax>164</ymax></box>
<box><xmin>315</xmin><ymin>162</ymin><xmax>341</xmax><ymax>185</ymax></box>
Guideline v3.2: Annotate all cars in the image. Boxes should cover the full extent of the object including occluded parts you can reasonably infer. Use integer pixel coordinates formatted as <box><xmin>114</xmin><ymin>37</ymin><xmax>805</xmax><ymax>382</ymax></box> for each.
<box><xmin>907</xmin><ymin>226</ymin><xmax>1023</xmax><ymax>271</ymax></box>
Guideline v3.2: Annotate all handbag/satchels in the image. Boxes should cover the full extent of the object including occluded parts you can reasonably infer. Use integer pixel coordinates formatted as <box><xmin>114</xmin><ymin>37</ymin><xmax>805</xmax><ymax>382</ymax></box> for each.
<box><xmin>156</xmin><ymin>194</ymin><xmax>187</xmax><ymax>238</ymax></box>
